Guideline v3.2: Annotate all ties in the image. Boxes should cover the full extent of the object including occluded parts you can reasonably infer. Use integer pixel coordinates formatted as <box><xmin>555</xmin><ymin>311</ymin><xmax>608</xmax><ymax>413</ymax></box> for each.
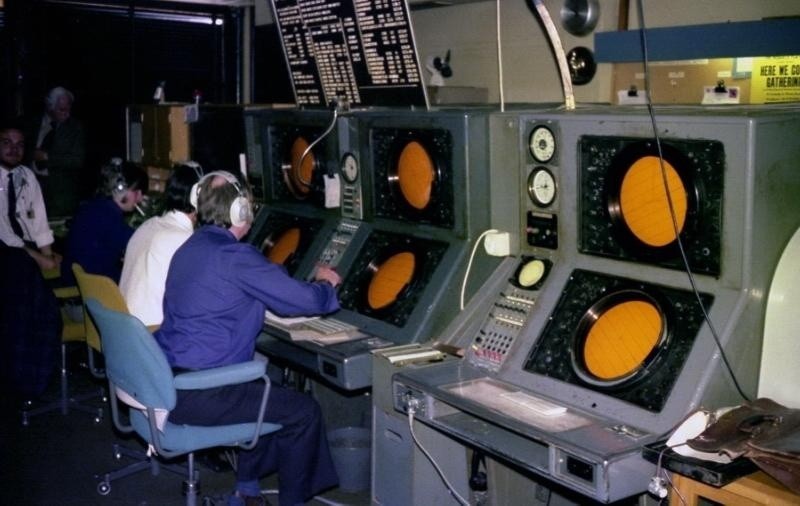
<box><xmin>6</xmin><ymin>172</ymin><xmax>24</xmax><ymax>239</ymax></box>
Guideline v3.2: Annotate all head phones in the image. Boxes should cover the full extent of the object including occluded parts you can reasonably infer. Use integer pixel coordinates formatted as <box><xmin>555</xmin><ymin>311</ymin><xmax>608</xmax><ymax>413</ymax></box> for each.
<box><xmin>188</xmin><ymin>169</ymin><xmax>254</xmax><ymax>230</ymax></box>
<box><xmin>181</xmin><ymin>159</ymin><xmax>205</xmax><ymax>180</ymax></box>
<box><xmin>109</xmin><ymin>154</ymin><xmax>128</xmax><ymax>199</ymax></box>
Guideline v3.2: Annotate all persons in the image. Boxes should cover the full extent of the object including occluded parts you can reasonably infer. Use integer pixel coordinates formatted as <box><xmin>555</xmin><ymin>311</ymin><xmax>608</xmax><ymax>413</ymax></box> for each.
<box><xmin>153</xmin><ymin>171</ymin><xmax>341</xmax><ymax>506</ymax></box>
<box><xmin>63</xmin><ymin>161</ymin><xmax>151</xmax><ymax>320</ymax></box>
<box><xmin>29</xmin><ymin>81</ymin><xmax>75</xmax><ymax>174</ymax></box>
<box><xmin>117</xmin><ymin>159</ymin><xmax>225</xmax><ymax>472</ymax></box>
<box><xmin>1</xmin><ymin>123</ymin><xmax>64</xmax><ymax>422</ymax></box>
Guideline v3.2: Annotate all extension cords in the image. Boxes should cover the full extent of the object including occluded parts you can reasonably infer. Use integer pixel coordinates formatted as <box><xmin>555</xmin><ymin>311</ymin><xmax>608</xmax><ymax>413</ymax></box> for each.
<box><xmin>323</xmin><ymin>172</ymin><xmax>339</xmax><ymax>210</ymax></box>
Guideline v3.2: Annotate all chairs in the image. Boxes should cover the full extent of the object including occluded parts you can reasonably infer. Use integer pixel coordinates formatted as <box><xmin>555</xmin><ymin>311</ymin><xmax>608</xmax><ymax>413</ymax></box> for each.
<box><xmin>70</xmin><ymin>262</ymin><xmax>161</xmax><ymax>434</ymax></box>
<box><xmin>81</xmin><ymin>298</ymin><xmax>282</xmax><ymax>506</ymax></box>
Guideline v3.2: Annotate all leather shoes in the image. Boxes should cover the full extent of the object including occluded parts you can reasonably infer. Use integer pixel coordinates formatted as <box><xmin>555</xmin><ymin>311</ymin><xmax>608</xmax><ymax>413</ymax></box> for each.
<box><xmin>229</xmin><ymin>490</ymin><xmax>273</xmax><ymax>506</ymax></box>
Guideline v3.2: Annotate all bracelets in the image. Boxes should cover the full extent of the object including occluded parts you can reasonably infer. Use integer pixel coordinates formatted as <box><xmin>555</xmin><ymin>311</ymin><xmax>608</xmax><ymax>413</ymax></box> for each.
<box><xmin>46</xmin><ymin>254</ymin><xmax>56</xmax><ymax>259</ymax></box>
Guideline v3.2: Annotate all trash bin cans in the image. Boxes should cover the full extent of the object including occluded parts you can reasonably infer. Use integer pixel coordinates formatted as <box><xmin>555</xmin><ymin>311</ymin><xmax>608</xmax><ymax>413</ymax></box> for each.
<box><xmin>326</xmin><ymin>425</ymin><xmax>372</xmax><ymax>495</ymax></box>
